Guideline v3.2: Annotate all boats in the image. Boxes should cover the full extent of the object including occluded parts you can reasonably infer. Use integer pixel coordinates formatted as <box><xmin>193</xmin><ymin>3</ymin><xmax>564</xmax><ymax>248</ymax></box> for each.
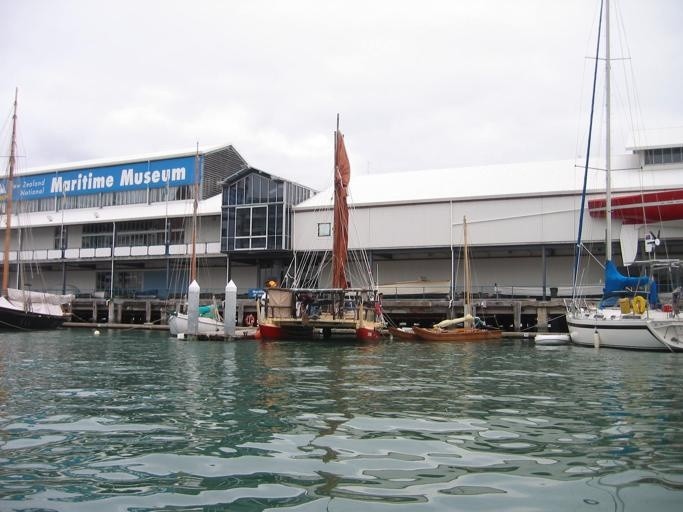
<box><xmin>534</xmin><ymin>333</ymin><xmax>570</xmax><ymax>345</ymax></box>
<box><xmin>384</xmin><ymin>319</ymin><xmax>421</xmax><ymax>342</ymax></box>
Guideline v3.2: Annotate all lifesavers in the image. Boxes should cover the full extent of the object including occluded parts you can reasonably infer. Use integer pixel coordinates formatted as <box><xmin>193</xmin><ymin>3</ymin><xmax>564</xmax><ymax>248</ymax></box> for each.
<box><xmin>632</xmin><ymin>296</ymin><xmax>645</xmax><ymax>314</ymax></box>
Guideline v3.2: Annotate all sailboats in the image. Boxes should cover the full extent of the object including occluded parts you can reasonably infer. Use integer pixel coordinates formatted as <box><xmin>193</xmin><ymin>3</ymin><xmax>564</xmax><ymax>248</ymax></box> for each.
<box><xmin>0</xmin><ymin>80</ymin><xmax>66</xmax><ymax>332</ymax></box>
<box><xmin>412</xmin><ymin>213</ymin><xmax>504</xmax><ymax>342</ymax></box>
<box><xmin>256</xmin><ymin>111</ymin><xmax>385</xmax><ymax>344</ymax></box>
<box><xmin>560</xmin><ymin>2</ymin><xmax>683</xmax><ymax>351</ymax></box>
<box><xmin>167</xmin><ymin>140</ymin><xmax>243</xmax><ymax>340</ymax></box>
